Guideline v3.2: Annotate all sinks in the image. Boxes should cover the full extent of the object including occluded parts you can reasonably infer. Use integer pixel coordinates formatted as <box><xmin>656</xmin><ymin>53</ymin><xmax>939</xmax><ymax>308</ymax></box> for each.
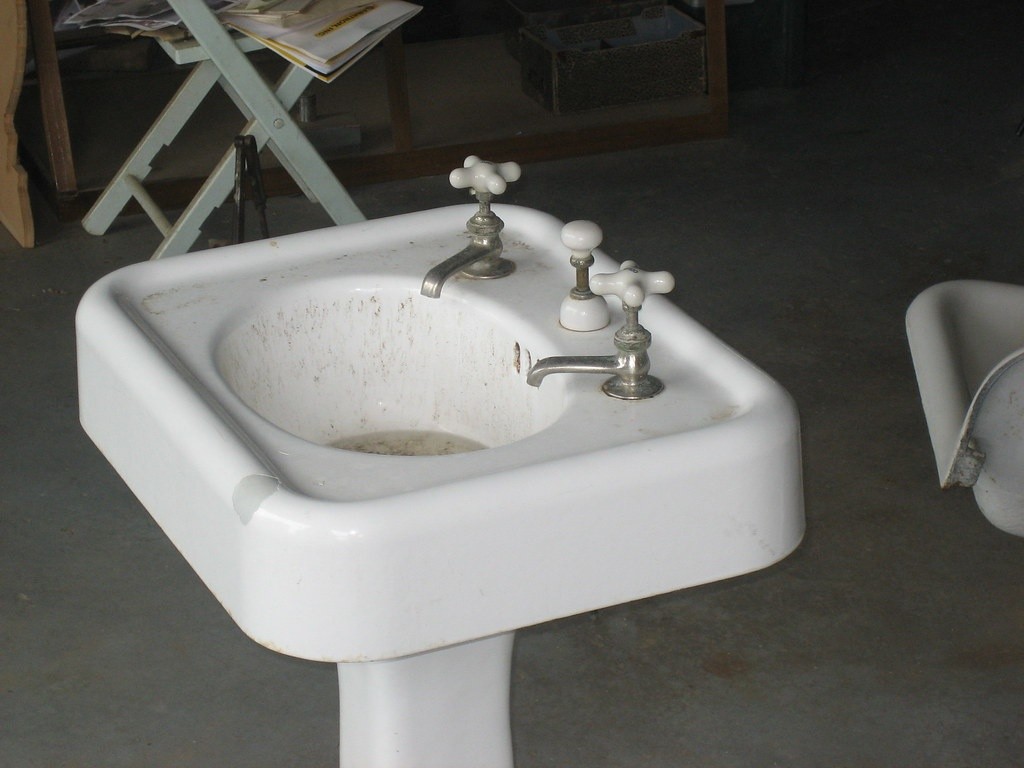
<box><xmin>69</xmin><ymin>193</ymin><xmax>819</xmax><ymax>666</ymax></box>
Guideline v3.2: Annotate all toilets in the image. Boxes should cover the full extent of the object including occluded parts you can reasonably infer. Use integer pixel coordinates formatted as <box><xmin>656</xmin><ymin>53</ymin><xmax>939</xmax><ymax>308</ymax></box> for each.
<box><xmin>904</xmin><ymin>278</ymin><xmax>1024</xmax><ymax>538</ymax></box>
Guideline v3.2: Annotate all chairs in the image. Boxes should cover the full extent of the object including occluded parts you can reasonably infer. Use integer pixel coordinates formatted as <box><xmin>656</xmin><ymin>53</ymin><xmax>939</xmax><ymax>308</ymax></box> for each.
<box><xmin>81</xmin><ymin>0</ymin><xmax>367</xmax><ymax>261</ymax></box>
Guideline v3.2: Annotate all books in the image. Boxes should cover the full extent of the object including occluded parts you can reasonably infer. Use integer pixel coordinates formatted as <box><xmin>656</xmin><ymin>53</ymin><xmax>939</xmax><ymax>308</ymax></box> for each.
<box><xmin>104</xmin><ymin>0</ymin><xmax>424</xmax><ymax>84</ymax></box>
<box><xmin>216</xmin><ymin>0</ymin><xmax>423</xmax><ymax>84</ymax></box>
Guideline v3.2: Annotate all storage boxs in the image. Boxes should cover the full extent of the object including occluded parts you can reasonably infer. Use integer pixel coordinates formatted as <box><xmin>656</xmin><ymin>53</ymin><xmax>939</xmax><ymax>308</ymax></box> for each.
<box><xmin>511</xmin><ymin>20</ymin><xmax>706</xmax><ymax>116</ymax></box>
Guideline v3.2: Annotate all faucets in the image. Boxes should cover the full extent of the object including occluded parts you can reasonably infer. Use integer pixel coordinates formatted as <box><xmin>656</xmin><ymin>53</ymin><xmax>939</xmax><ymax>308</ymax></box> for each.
<box><xmin>420</xmin><ymin>154</ymin><xmax>521</xmax><ymax>300</ymax></box>
<box><xmin>526</xmin><ymin>259</ymin><xmax>676</xmax><ymax>404</ymax></box>
